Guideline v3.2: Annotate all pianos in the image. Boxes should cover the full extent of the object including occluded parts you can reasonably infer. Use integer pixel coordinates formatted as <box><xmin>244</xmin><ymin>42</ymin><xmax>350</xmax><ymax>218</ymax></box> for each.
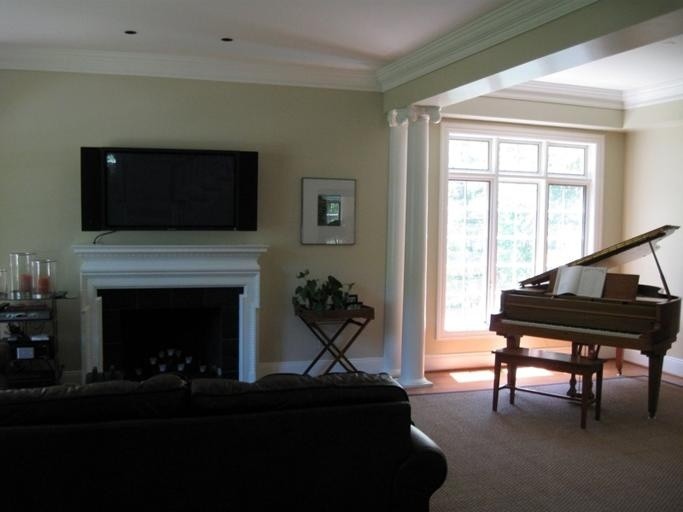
<box><xmin>489</xmin><ymin>225</ymin><xmax>681</xmax><ymax>420</ymax></box>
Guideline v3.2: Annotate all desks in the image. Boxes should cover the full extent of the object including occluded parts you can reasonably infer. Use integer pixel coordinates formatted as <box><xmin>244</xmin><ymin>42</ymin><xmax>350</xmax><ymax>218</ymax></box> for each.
<box><xmin>293</xmin><ymin>305</ymin><xmax>374</xmax><ymax>377</ymax></box>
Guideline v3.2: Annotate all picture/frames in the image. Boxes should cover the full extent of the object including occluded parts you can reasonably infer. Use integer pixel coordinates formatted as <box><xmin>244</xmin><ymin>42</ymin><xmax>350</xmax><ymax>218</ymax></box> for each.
<box><xmin>299</xmin><ymin>177</ymin><xmax>357</xmax><ymax>246</ymax></box>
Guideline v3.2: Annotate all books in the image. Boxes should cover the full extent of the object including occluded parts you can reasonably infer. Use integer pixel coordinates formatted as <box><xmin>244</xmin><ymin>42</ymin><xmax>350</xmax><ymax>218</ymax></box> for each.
<box><xmin>550</xmin><ymin>265</ymin><xmax>608</xmax><ymax>299</ymax></box>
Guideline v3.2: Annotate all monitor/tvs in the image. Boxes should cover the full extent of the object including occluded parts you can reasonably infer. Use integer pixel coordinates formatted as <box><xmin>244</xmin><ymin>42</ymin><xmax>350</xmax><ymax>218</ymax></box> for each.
<box><xmin>79</xmin><ymin>147</ymin><xmax>259</xmax><ymax>231</ymax></box>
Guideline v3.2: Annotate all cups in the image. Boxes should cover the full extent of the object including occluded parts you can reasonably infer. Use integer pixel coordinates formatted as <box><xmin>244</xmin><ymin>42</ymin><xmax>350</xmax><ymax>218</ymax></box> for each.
<box><xmin>0</xmin><ymin>251</ymin><xmax>57</xmax><ymax>300</ymax></box>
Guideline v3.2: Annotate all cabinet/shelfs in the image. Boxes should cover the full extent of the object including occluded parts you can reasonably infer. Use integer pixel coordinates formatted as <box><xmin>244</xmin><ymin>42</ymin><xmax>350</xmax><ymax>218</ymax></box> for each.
<box><xmin>0</xmin><ymin>297</ymin><xmax>58</xmax><ymax>371</ymax></box>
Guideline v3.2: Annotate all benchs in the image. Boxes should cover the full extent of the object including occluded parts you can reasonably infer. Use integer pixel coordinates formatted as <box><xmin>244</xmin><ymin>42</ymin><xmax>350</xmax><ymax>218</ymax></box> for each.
<box><xmin>489</xmin><ymin>346</ymin><xmax>609</xmax><ymax>430</ymax></box>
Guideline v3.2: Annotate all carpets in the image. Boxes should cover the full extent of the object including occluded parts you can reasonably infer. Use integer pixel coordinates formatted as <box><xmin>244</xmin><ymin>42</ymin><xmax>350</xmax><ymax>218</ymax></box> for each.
<box><xmin>408</xmin><ymin>373</ymin><xmax>683</xmax><ymax>512</ymax></box>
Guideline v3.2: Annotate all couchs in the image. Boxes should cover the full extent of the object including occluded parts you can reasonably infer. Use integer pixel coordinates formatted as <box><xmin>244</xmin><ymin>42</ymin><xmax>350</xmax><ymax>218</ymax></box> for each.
<box><xmin>0</xmin><ymin>369</ymin><xmax>447</xmax><ymax>510</ymax></box>
